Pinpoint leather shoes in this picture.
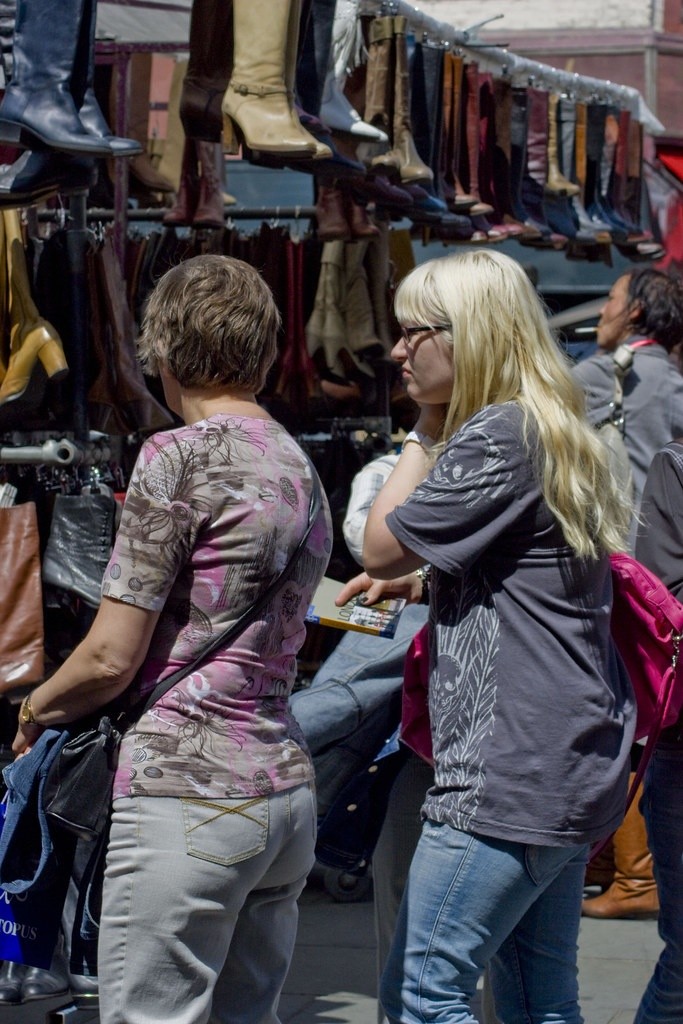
[0,961,29,1004]
[20,951,68,1002]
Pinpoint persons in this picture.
[14,253,336,1024]
[335,248,637,1024]
[570,265,683,495]
[635,438,683,1024]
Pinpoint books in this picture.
[306,576,407,639]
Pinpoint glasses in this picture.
[401,324,452,345]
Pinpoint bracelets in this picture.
[416,568,429,606]
[401,431,436,453]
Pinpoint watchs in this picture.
[22,697,37,724]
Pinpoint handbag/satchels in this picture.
[0,502,46,696]
[43,712,125,839]
[604,550,683,741]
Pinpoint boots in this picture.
[575,772,659,920]
[0,0,668,440]
[41,491,115,610]
